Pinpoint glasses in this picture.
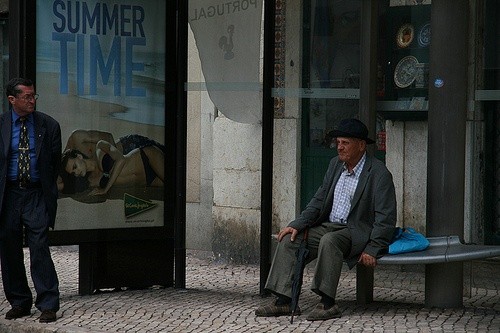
[14,93,39,100]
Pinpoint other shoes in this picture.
[305,302,342,320]
[39,311,56,322]
[255,297,301,316]
[5,306,31,319]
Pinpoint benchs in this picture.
[271,235,500,309]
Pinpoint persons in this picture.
[0,78,63,322]
[57,130,165,197]
[255,119,397,320]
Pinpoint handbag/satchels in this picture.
[385,227,430,254]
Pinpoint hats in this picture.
[334,119,376,144]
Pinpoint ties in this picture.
[17,117,32,186]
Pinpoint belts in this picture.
[7,182,40,190]
